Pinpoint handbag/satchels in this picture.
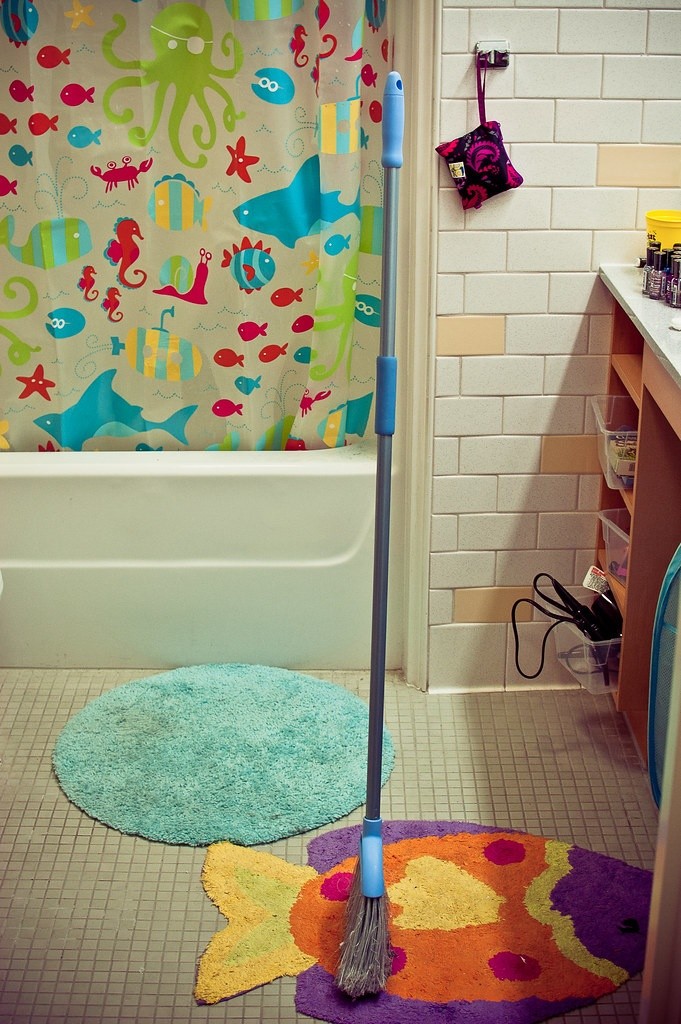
[435,51,523,210]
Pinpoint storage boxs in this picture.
[589,396,641,492]
[545,591,622,696]
[596,508,636,589]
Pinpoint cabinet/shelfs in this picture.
[594,262,681,772]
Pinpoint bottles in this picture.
[642,241,681,308]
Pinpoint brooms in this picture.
[329,69,406,998]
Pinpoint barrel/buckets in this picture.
[644,209,680,251]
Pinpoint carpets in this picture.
[50,662,655,1023]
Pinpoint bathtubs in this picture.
[1,427,407,671]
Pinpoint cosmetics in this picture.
[636,241,681,308]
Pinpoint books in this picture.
[608,435,636,476]
